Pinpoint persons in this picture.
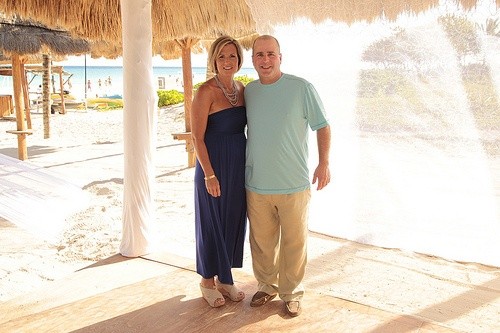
[189,33,248,308]
[38,74,186,98]
[240,33,332,317]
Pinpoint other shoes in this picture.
[250,291,272,306]
[285,301,302,317]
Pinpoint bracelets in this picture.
[203,174,216,180]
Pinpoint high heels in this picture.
[216,278,245,302]
[199,283,225,307]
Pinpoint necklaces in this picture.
[213,73,240,106]
